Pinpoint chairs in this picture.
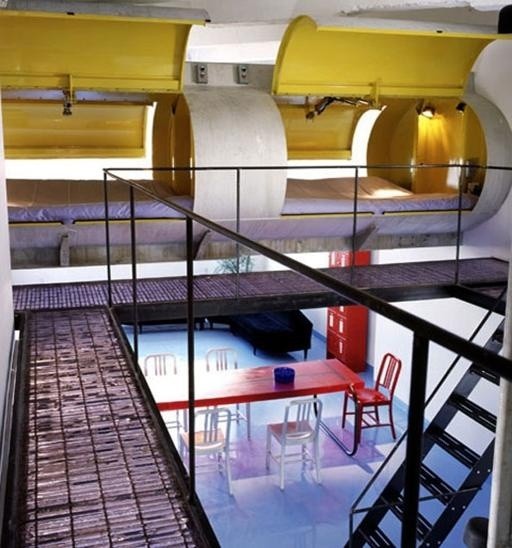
[143,347,402,496]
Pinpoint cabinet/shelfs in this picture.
[326,249,371,373]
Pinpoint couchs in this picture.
[230,310,314,362]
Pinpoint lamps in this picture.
[455,102,469,114]
[415,101,436,119]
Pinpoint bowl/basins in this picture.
[274,367,295,384]
